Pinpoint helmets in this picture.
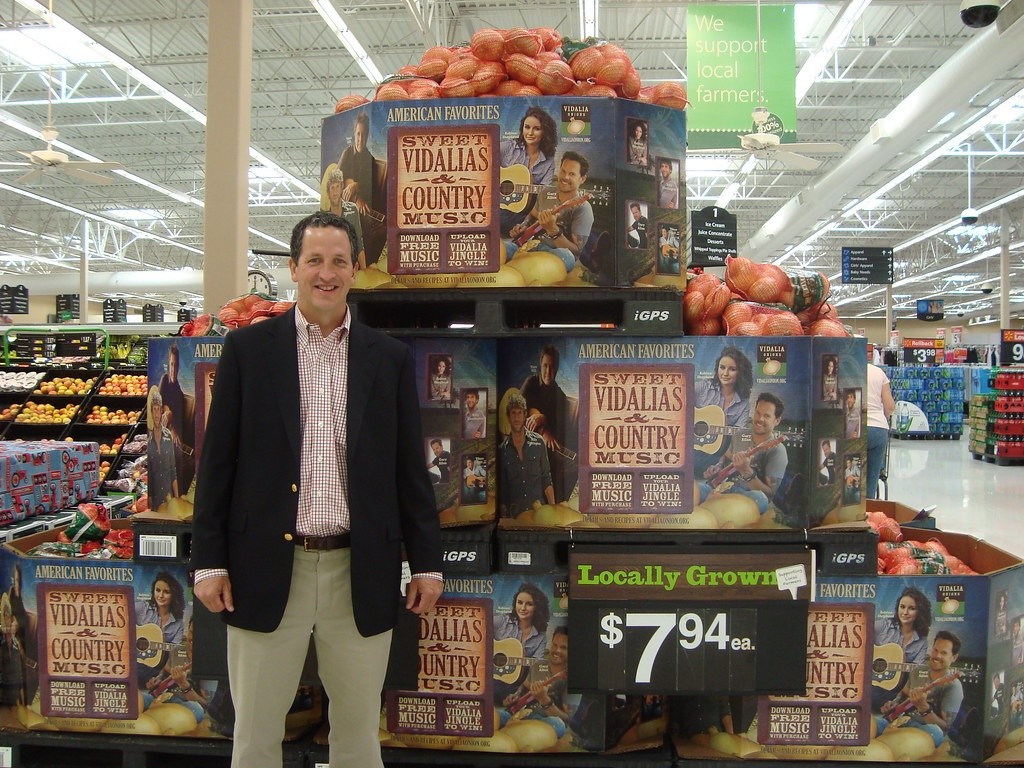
[506,393,528,417]
[326,170,344,190]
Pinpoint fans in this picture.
[11,0,122,186]
[686,0,845,169]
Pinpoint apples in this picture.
[0,373,148,486]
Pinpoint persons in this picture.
[192,211,445,768]
[865,362,895,499]
[0,106,1024,768]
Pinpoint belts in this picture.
[295,532,351,552]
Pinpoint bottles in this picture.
[896,403,901,428]
[901,403,909,429]
[882,366,965,433]
[969,366,1024,455]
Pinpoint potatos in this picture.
[180,293,296,336]
[683,256,849,339]
[861,510,982,575]
[333,29,685,108]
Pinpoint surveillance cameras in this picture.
[961,208,979,225]
[980,283,993,293]
[959,0,1001,28]
[957,311,964,317]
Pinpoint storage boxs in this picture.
[0,97,1024,768]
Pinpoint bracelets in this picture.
[548,229,562,240]
[919,705,932,716]
[541,699,554,708]
[181,684,192,693]
[742,470,757,482]
